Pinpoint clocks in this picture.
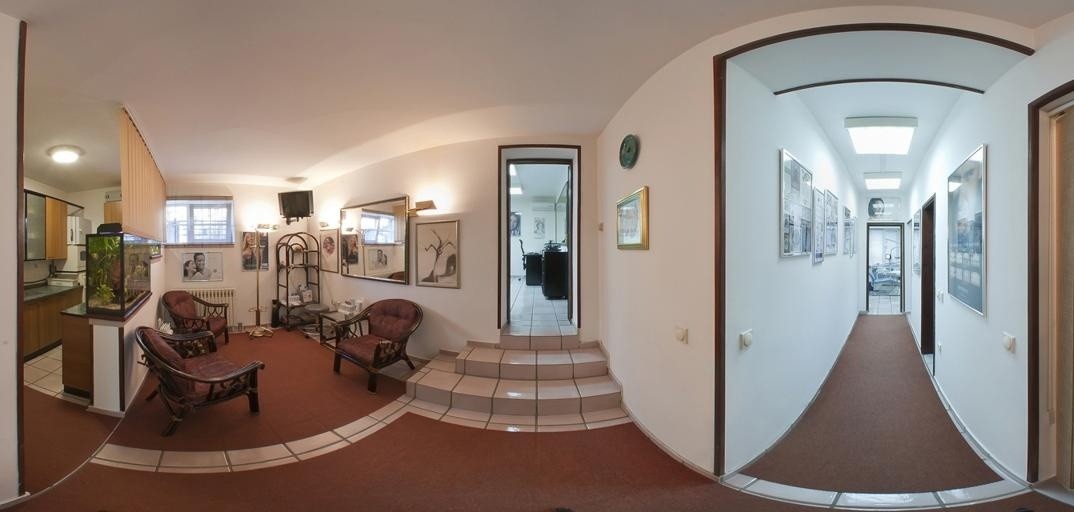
[618,135,638,169]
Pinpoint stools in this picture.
[300,303,329,339]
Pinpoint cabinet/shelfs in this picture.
[525,250,567,299]
[243,230,320,340]
[22,189,93,400]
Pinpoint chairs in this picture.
[333,298,423,394]
[134,292,265,437]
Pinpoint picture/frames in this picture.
[362,244,404,277]
[945,143,986,319]
[779,148,855,266]
[910,209,921,280]
[318,229,338,273]
[338,195,409,285]
[615,185,649,251]
[180,251,223,282]
[413,219,460,289]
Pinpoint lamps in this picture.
[509,164,522,195]
[408,200,436,218]
[843,116,918,190]
[46,145,83,164]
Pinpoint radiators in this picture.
[170,288,235,331]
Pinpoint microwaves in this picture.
[53,245,87,273]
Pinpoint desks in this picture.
[319,310,356,351]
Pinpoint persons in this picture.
[370,249,392,268]
[241,233,261,268]
[183,253,213,280]
[342,235,361,265]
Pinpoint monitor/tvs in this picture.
[278,190,314,219]
